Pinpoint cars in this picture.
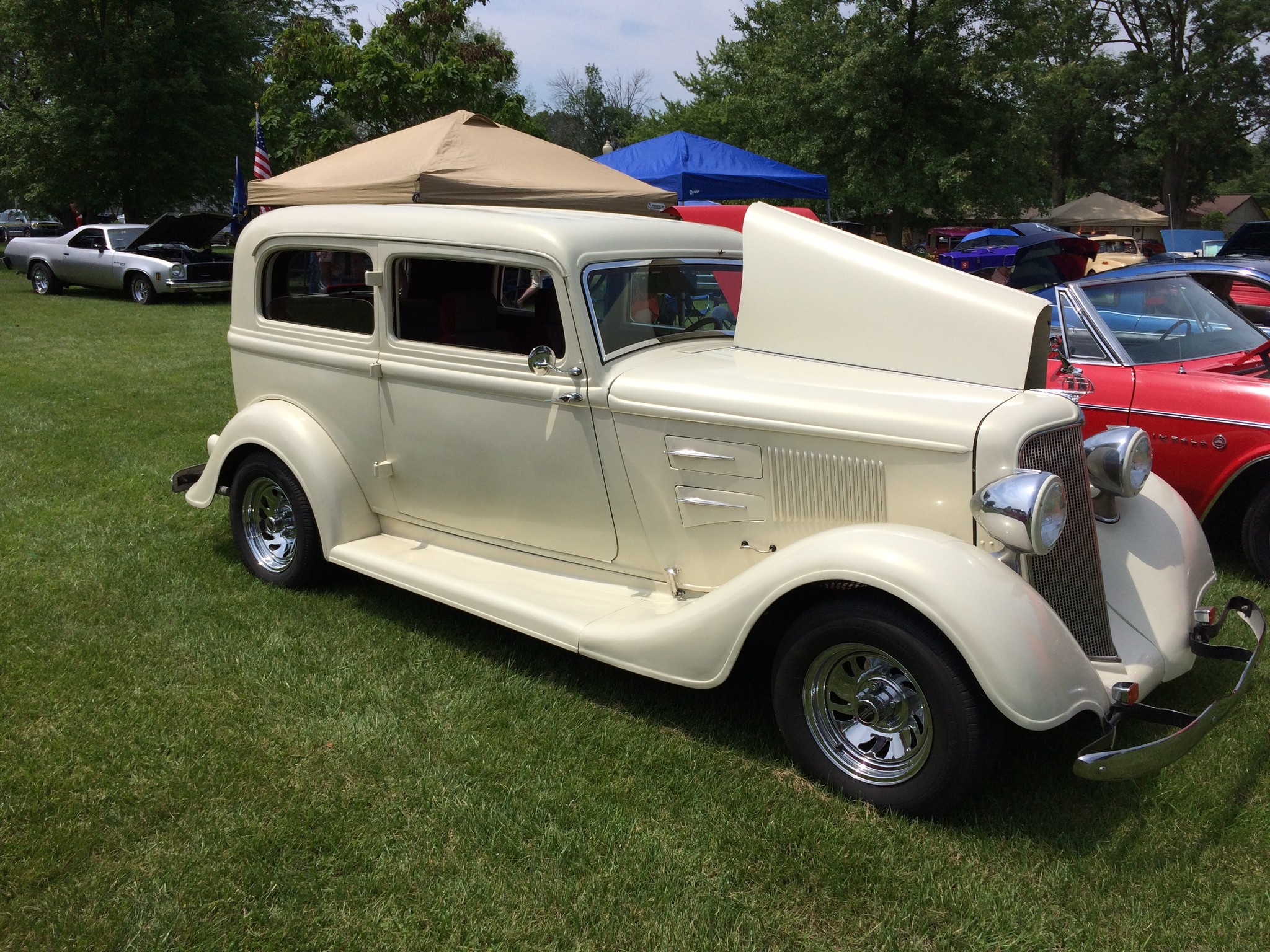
[0,204,232,304]
[167,206,1264,809]
[657,206,1270,577]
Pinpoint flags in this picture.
[229,159,252,237]
[253,112,273,214]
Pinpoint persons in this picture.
[709,293,735,328]
[516,269,557,314]
[631,267,692,325]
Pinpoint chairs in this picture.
[973,272,990,280]
[591,284,732,330]
[89,236,106,250]
[438,286,507,353]
[533,289,566,362]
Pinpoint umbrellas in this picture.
[949,227,1020,251]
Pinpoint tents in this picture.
[1029,191,1169,251]
[247,107,681,221]
[590,129,834,225]
